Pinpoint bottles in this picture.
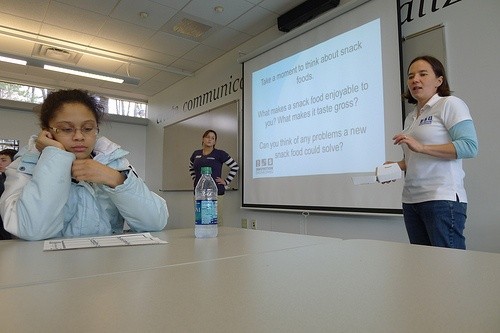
[195,167,218,239]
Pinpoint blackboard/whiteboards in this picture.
[157,98,239,192]
[402,21,448,117]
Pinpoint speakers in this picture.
[277,0,340,32]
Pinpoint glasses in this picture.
[45,124,100,137]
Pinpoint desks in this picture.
[0,227,500,333]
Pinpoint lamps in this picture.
[44,64,124,83]
[0,56,27,65]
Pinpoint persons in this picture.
[375,56,479,250]
[0,89,169,241]
[189,130,239,227]
[0,149,21,240]
